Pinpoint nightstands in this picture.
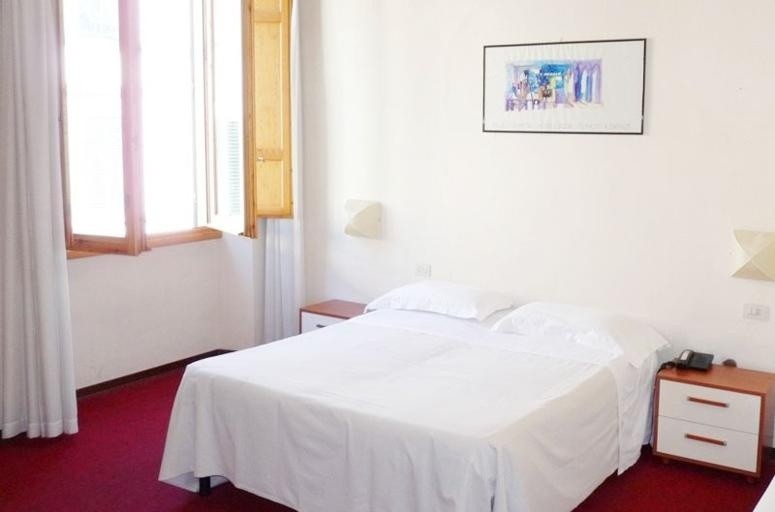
[299,299,367,332]
[653,358,774,484]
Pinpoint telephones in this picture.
[676,350,713,371]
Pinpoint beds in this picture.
[174,282,659,510]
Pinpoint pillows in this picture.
[366,279,665,367]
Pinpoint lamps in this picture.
[342,197,383,239]
[724,229,775,280]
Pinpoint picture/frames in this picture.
[482,38,646,135]
